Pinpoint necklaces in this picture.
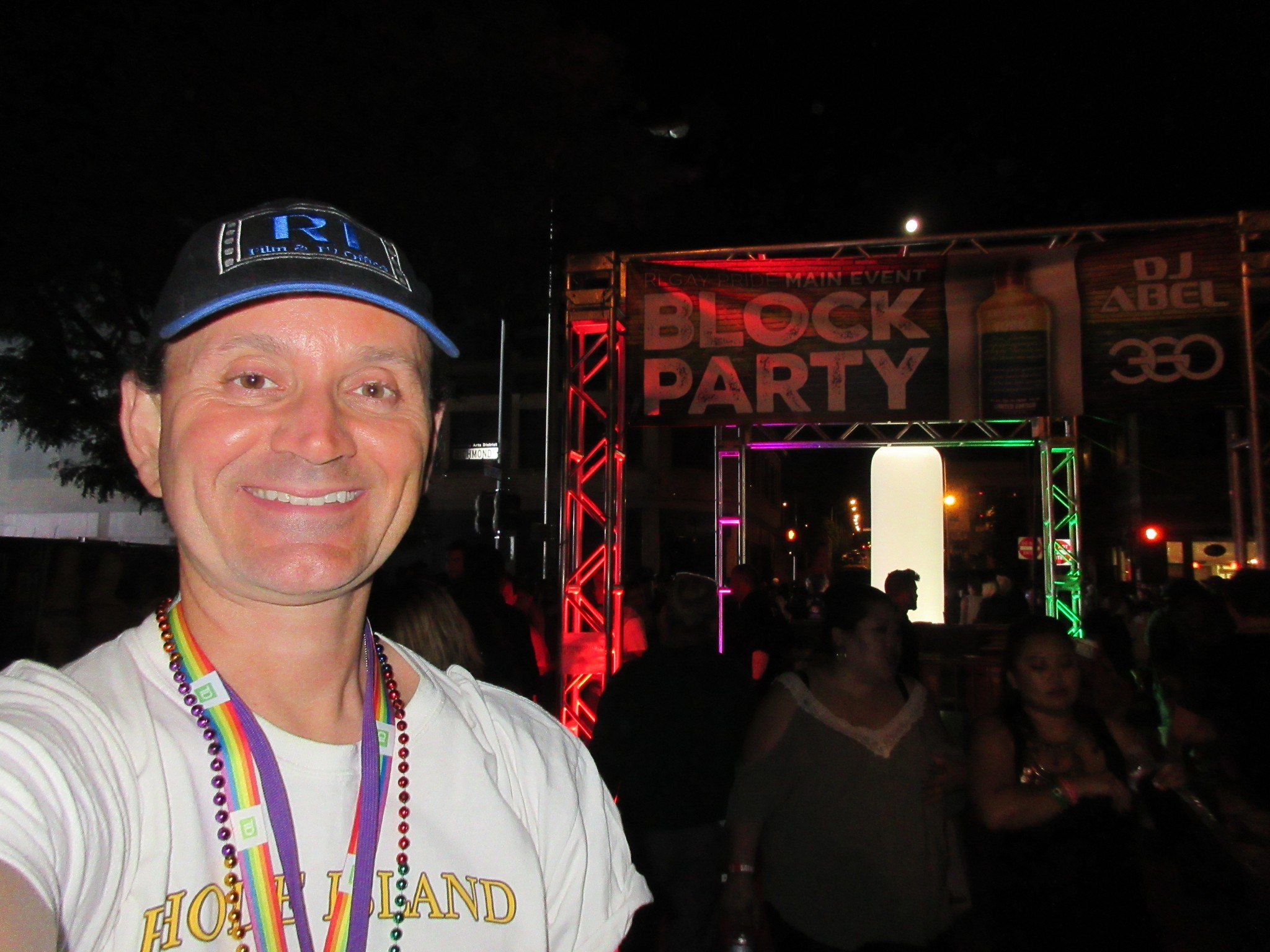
[1029,726,1084,768]
[151,590,412,952]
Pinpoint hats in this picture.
[154,197,460,360]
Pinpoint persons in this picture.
[0,195,659,952]
[1169,561,1270,952]
[716,584,980,952]
[351,532,1238,754]
[963,614,1197,952]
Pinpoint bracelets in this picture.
[725,861,758,877]
[1038,770,1079,810]
